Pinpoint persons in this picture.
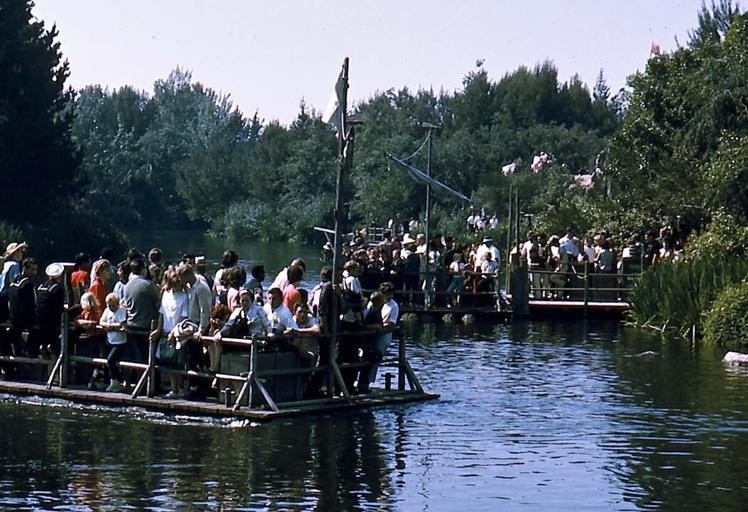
[500,149,613,199]
[1,238,402,406]
[340,206,700,310]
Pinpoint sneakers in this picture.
[87,378,207,402]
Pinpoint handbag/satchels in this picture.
[220,317,250,339]
[155,338,179,368]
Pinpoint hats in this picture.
[45,262,65,279]
[481,235,493,246]
[401,237,416,245]
[3,242,30,261]
